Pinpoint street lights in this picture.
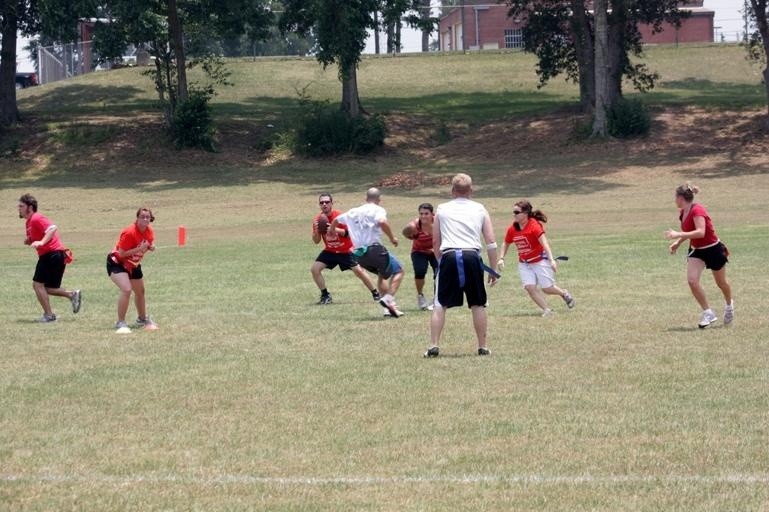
[714,26,722,42]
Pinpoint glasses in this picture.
[513,211,523,215]
[320,201,330,204]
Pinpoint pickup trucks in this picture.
[15,72,38,90]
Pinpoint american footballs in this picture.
[403,224,417,235]
[318,213,329,233]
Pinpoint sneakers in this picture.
[116,320,130,329]
[373,292,434,318]
[478,348,491,355]
[71,289,81,313]
[135,317,159,327]
[698,313,718,328]
[317,295,332,304]
[723,300,734,324]
[561,289,575,308]
[424,347,439,358]
[35,313,57,323]
[542,308,555,317]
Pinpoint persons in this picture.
[328,187,406,318]
[402,202,441,312]
[310,192,382,306]
[664,182,736,329]
[17,193,82,322]
[421,172,500,359]
[105,207,160,334]
[495,200,576,318]
